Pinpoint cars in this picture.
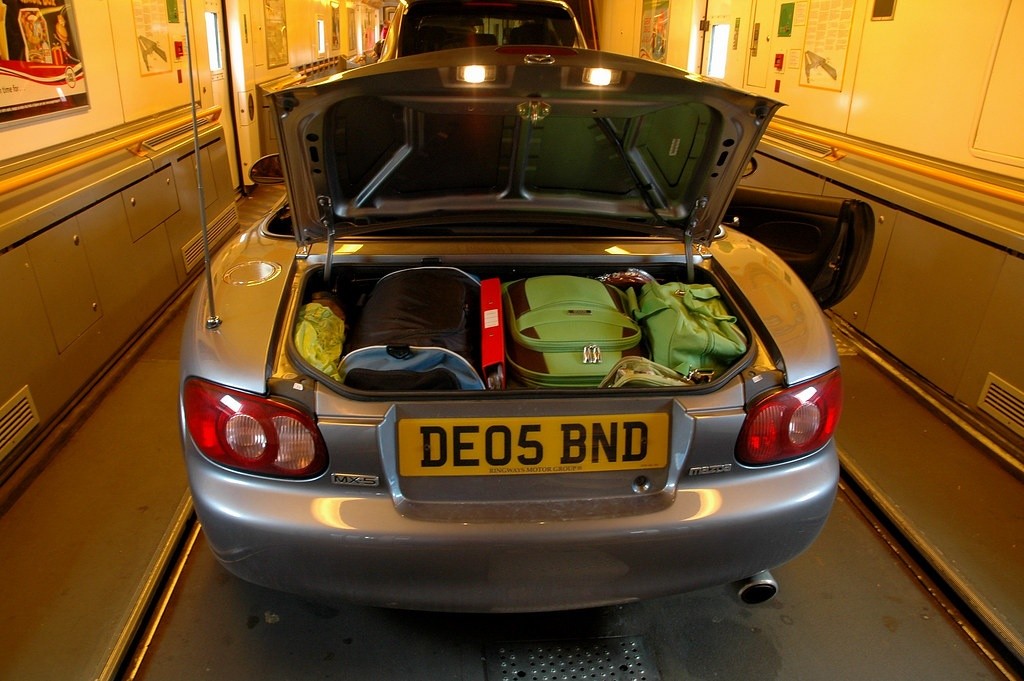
[178,44,875,647]
[374,1,588,89]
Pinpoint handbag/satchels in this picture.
[337,264,486,391]
[595,356,697,388]
[623,277,749,384]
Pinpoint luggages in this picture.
[501,275,650,389]
